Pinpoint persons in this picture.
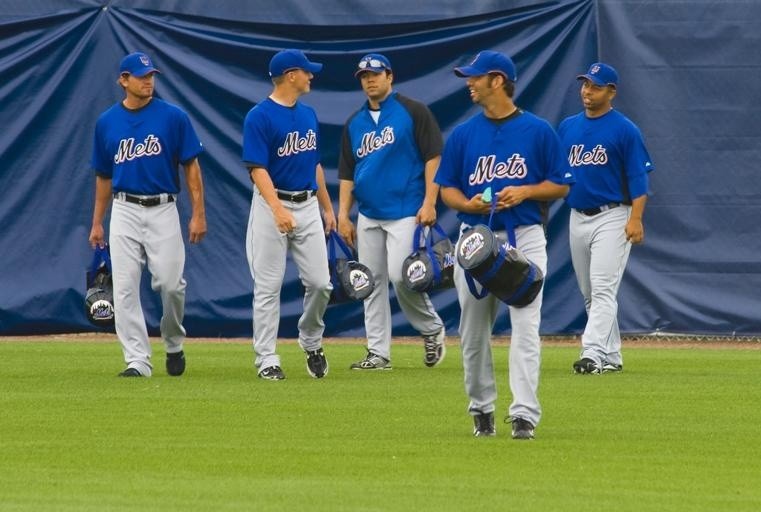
[433,50,576,441]
[243,49,336,381]
[338,53,445,370]
[556,63,655,373]
[89,54,208,377]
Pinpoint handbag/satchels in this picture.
[84,245,114,326]
[457,195,544,308]
[303,231,375,305]
[401,222,456,292]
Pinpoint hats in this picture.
[454,51,517,82]
[268,49,323,76]
[354,54,392,78]
[119,52,161,78]
[578,62,620,89]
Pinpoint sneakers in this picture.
[298,339,329,379]
[574,358,601,374]
[260,366,285,380]
[470,408,496,438]
[424,327,445,366]
[350,352,393,371]
[118,368,141,377]
[504,415,534,439]
[166,350,186,376]
[603,362,622,372]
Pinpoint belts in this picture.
[574,201,621,216]
[275,190,316,203]
[114,193,174,207]
[488,225,519,231]
